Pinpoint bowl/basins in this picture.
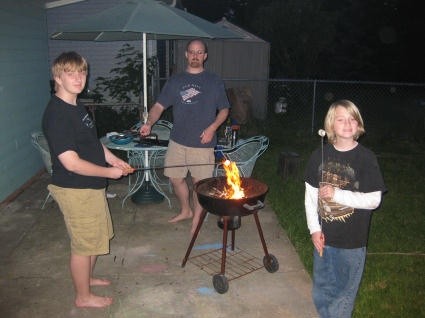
[110,134,133,144]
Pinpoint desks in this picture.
[98,136,172,209]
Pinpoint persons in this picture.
[302,99,387,318]
[42,52,134,308]
[140,39,231,236]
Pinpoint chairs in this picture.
[214,135,270,179]
[126,120,173,194]
[31,133,54,208]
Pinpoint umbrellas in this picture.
[49,0,245,182]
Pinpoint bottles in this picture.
[224,119,238,147]
